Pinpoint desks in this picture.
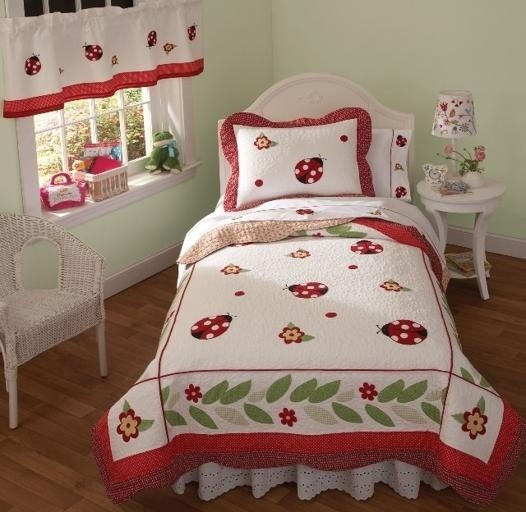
[416,175,505,301]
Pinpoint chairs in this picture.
[0,213,108,427]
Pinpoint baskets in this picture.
[71,164,128,203]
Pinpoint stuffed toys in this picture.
[143,129,184,176]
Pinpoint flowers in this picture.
[436,144,487,175]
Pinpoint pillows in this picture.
[220,106,414,212]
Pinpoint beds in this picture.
[92,70,525,508]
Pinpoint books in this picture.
[445,250,493,277]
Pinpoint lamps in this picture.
[432,91,477,182]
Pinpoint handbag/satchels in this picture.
[39,172,88,211]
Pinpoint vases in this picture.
[465,172,485,187]
